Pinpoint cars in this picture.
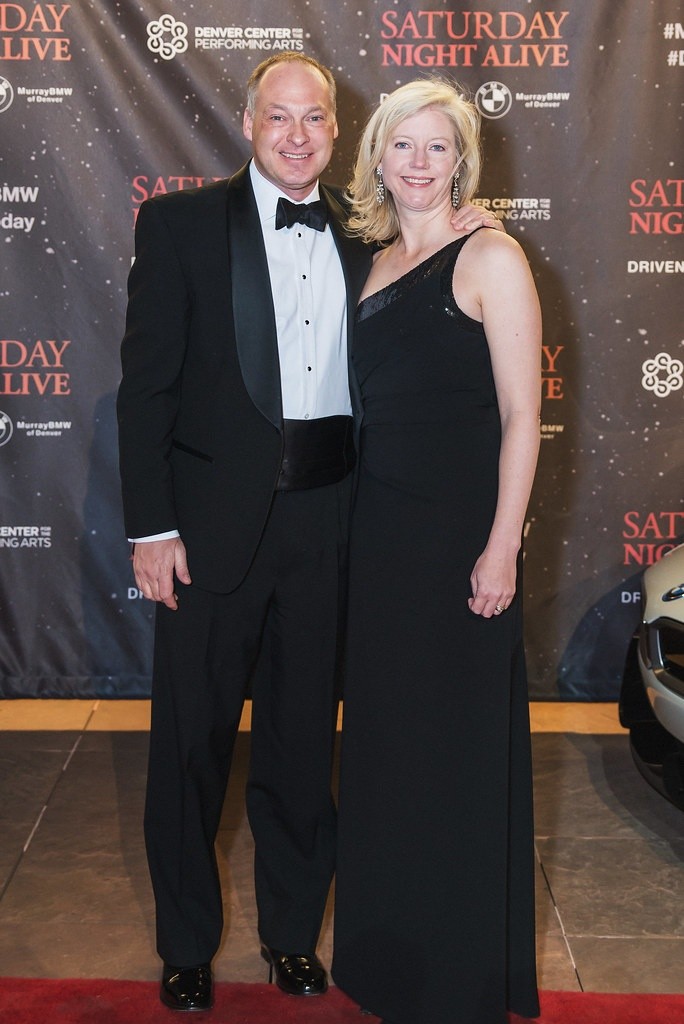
[637,542,684,744]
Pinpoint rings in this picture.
[495,605,504,612]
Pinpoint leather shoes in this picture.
[160,961,215,1012]
[261,943,329,996]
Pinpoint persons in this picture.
[116,54,507,1015]
[331,69,543,1024]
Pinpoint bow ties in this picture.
[275,196,327,232]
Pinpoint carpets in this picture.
[0,980,683,1023]
